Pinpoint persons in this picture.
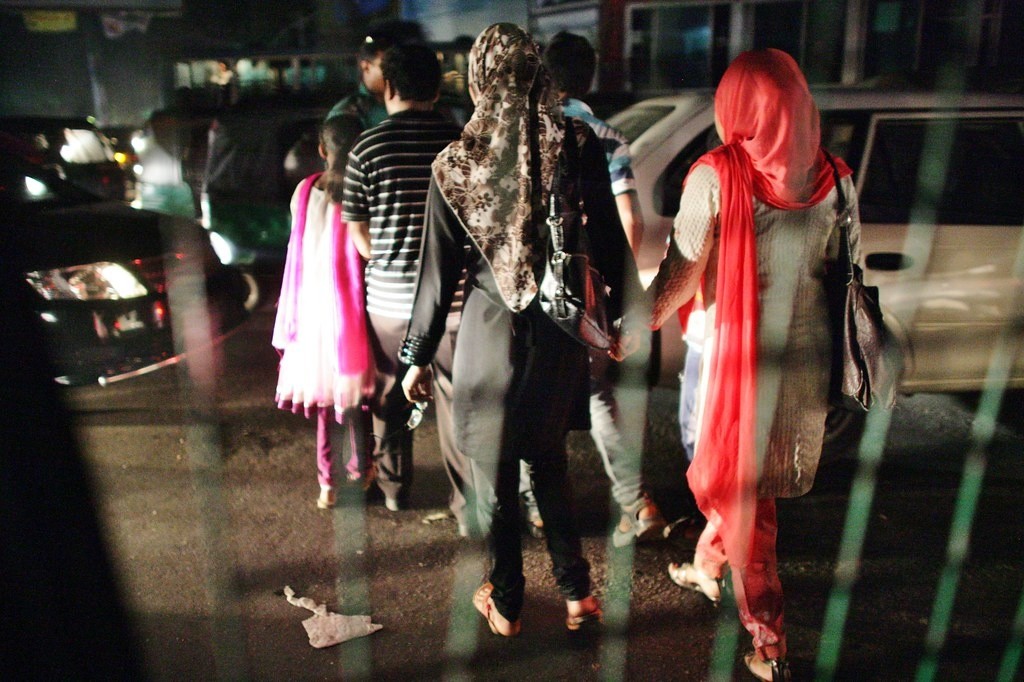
[435,70,474,126]
[527,31,664,551]
[610,46,862,682]
[399,24,644,643]
[321,39,393,128]
[342,46,476,541]
[273,112,374,511]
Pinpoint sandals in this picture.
[614,496,664,548]
[522,499,546,538]
[743,652,792,682]
[669,562,727,602]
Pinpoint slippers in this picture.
[564,606,603,630]
[474,582,520,637]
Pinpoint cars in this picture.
[600,75,1024,473]
[0,127,260,395]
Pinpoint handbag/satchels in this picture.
[822,149,898,413]
[540,116,615,350]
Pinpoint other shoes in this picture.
[348,473,373,492]
[317,487,336,510]
[376,478,407,511]
